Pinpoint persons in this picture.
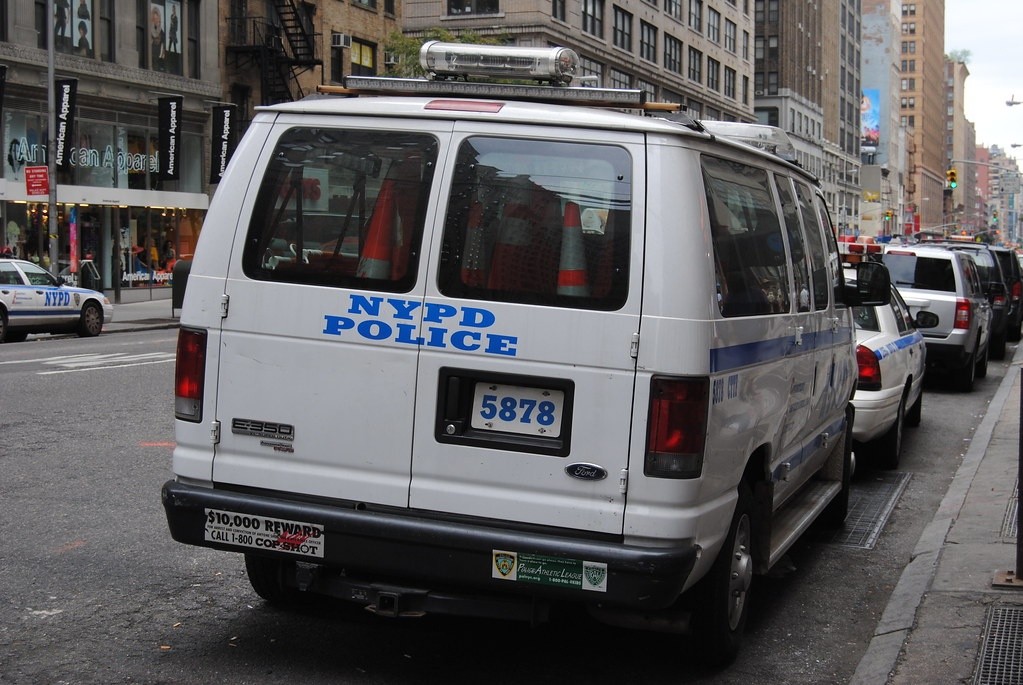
[164,240,176,271]
[151,239,161,270]
[53,0,69,44]
[168,5,177,54]
[4,138,26,182]
[131,245,146,274]
[78,21,89,49]
[151,7,164,58]
[77,0,89,19]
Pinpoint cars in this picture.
[837,261,939,472]
[0,258,114,343]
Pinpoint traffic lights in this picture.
[946,168,958,188]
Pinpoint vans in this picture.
[158,39,894,674]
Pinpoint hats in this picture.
[762,279,770,283]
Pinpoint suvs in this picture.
[882,236,1023,392]
